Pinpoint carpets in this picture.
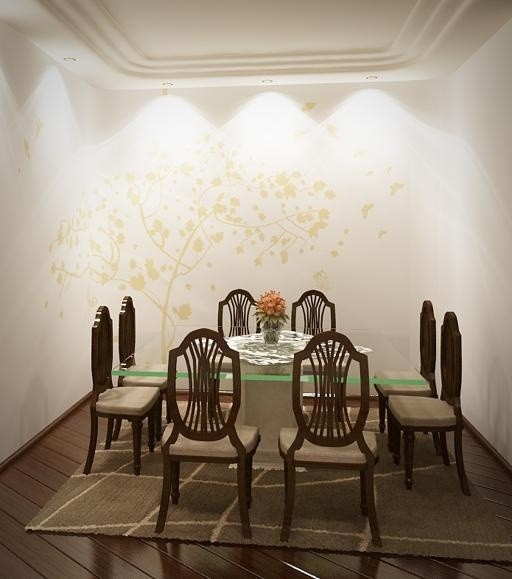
[25,399,512,563]
